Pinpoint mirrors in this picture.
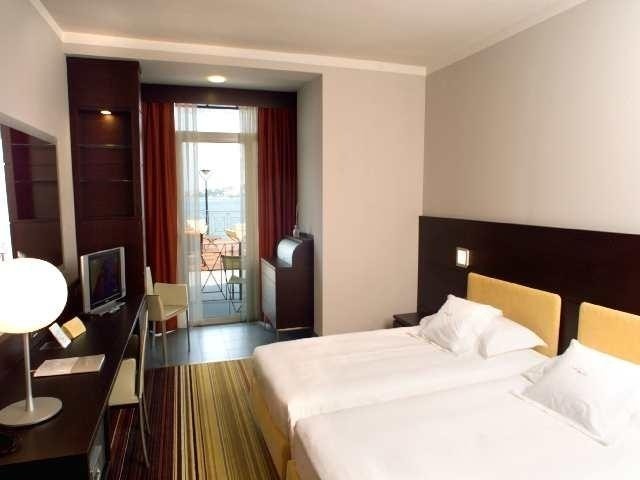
[2,112,64,277]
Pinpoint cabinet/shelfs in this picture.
[0,285,149,480]
[261,235,314,343]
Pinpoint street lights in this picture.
[200,170,212,235]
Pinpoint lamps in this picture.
[0,257,68,428]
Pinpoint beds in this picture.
[248,273,640,480]
[248,273,565,480]
[291,300,640,480]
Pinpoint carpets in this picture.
[101,357,282,480]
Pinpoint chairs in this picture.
[221,254,246,314]
[109,307,151,469]
[143,267,190,368]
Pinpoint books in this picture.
[33,354,106,379]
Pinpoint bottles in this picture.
[292,223,301,238]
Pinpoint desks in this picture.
[199,236,242,300]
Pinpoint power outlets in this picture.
[455,248,469,268]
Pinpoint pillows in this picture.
[417,294,503,349]
[529,338,639,447]
[478,317,546,357]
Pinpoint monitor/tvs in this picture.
[80,247,126,316]
